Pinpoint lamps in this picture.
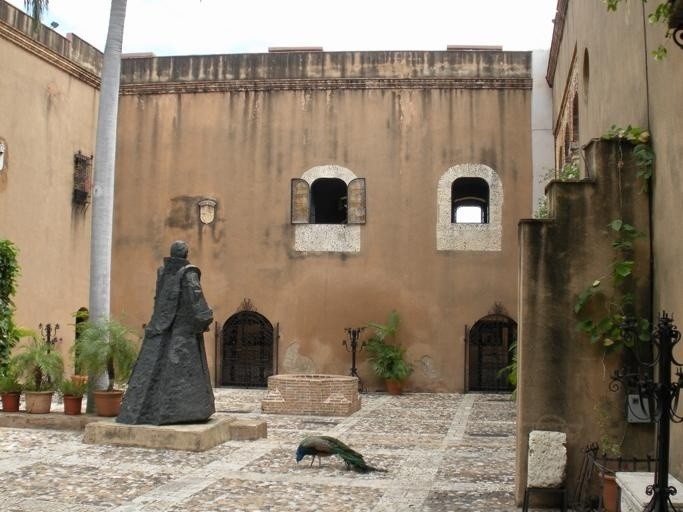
[50,21,58,30]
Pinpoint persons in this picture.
[116,240,215,425]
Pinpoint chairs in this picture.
[523,417,568,512]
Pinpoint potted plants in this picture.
[9,328,64,414]
[576,399,652,512]
[69,310,142,416]
[60,379,88,414]
[366,312,414,394]
[0,369,23,411]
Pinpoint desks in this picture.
[614,471,683,512]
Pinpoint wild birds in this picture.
[295,436,389,475]
